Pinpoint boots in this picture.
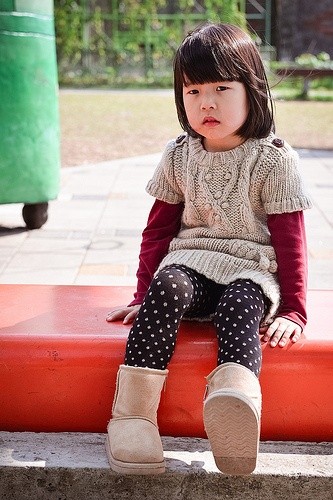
[106,365,169,475]
[203,362,261,475]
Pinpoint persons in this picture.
[105,22,311,475]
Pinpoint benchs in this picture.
[0,284,333,442]
[270,67,333,97]
